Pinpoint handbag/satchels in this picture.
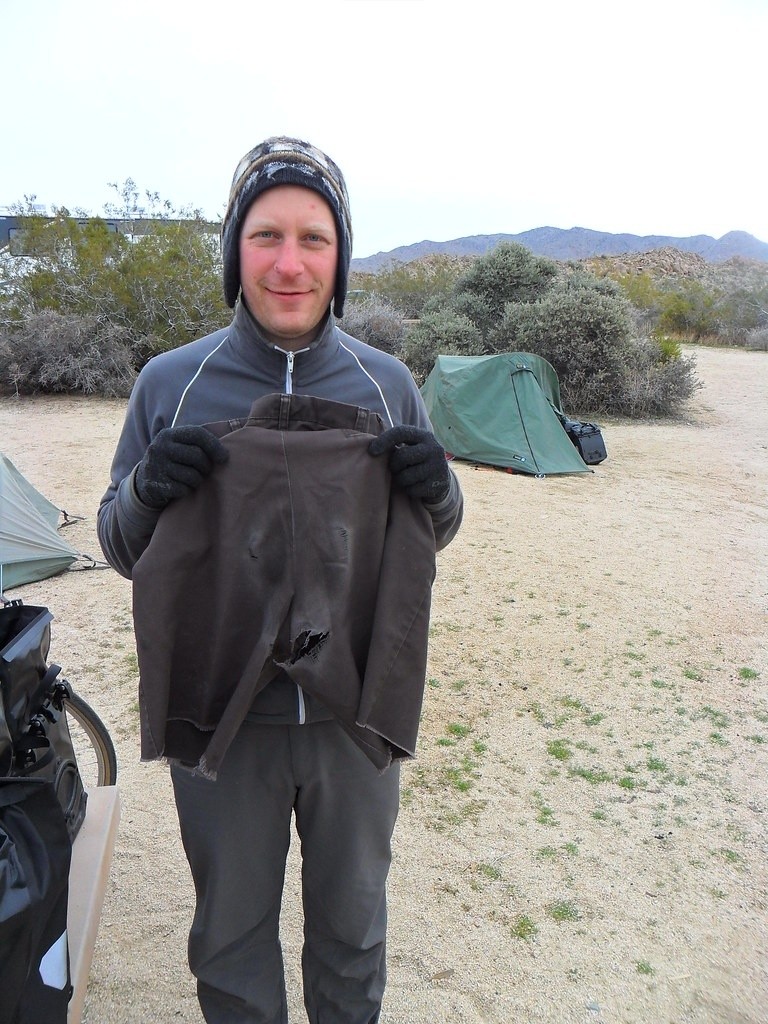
[0,600,88,844]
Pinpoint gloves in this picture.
[368,423,450,504]
[135,425,230,509]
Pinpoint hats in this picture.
[221,138,352,319]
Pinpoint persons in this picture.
[96,136,464,1024]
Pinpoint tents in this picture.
[419,351,594,476]
[0,453,79,591]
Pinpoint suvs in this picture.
[0,597,121,1024]
